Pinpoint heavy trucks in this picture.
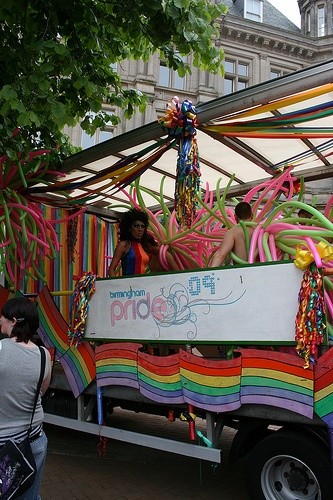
[0,188,333,500]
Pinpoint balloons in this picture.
[107,166,333,347]
[0,127,92,296]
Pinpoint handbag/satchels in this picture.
[0,438,37,500]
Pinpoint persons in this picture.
[203,200,265,269]
[108,206,152,276]
[0,296,52,499]
